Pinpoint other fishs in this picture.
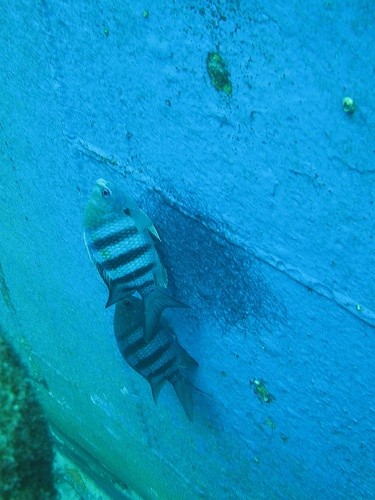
[113,296,214,424]
[81,179,195,345]
[206,51,234,96]
